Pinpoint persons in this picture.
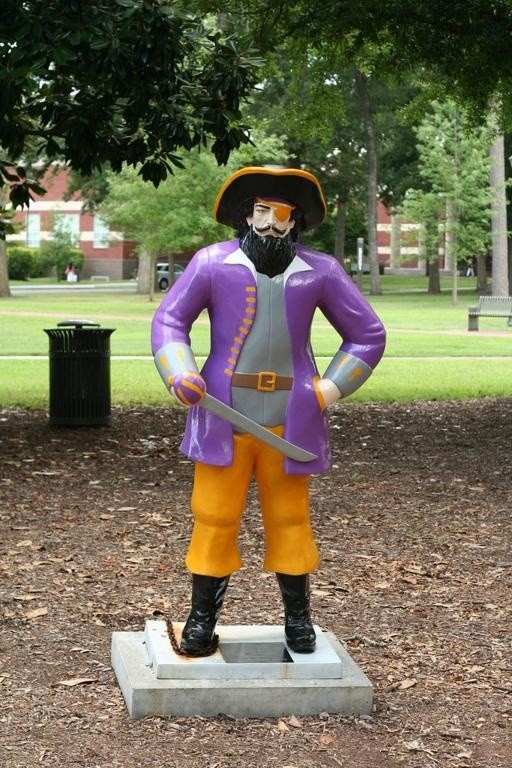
[466,259,476,278]
[148,161,389,657]
[64,261,75,284]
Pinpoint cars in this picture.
[156,262,185,289]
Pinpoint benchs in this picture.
[468,296,512,331]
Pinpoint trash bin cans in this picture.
[42,319,116,428]
